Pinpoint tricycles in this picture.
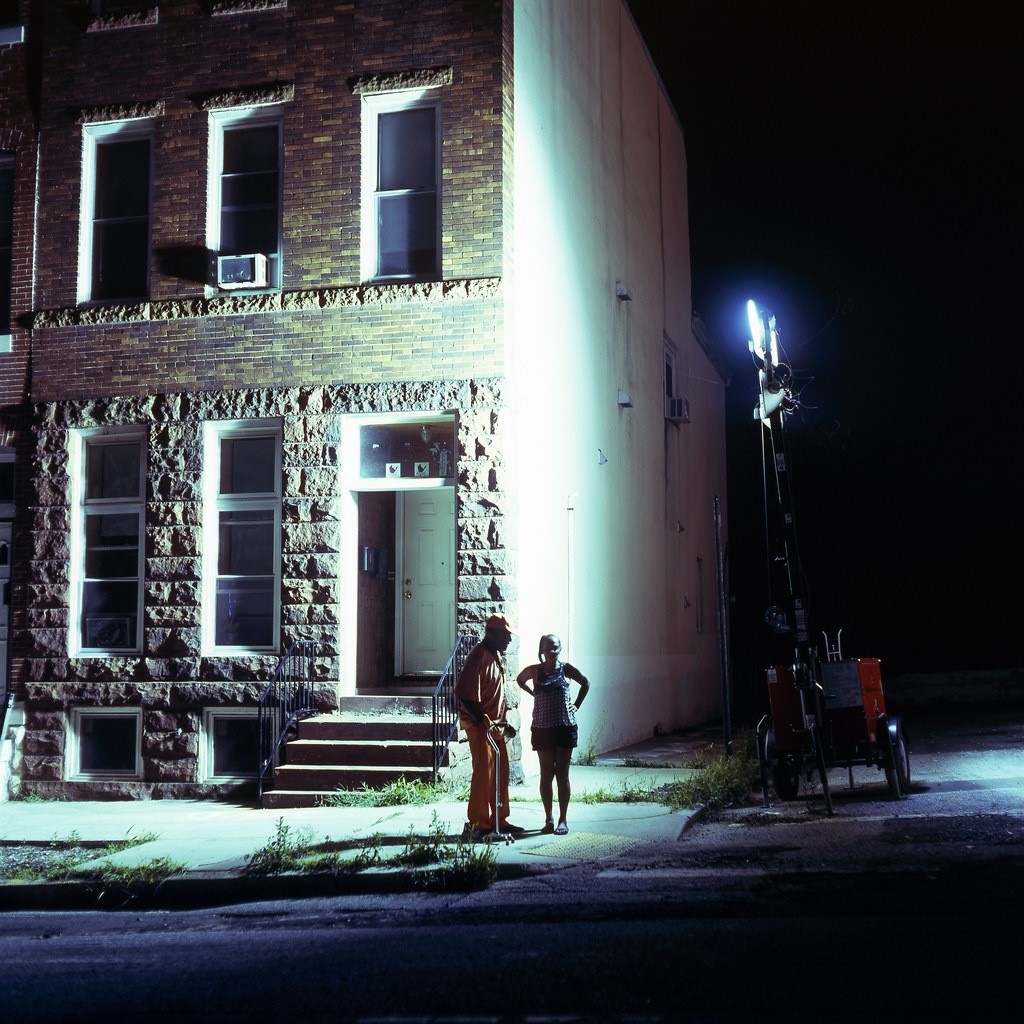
[755,626,915,801]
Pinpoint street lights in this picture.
[746,298,831,797]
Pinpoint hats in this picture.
[486,613,515,634]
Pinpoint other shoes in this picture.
[492,823,525,835]
[462,823,501,841]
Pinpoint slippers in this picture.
[542,822,553,833]
[555,823,569,835]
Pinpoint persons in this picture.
[516,634,591,833]
[454,613,524,838]
